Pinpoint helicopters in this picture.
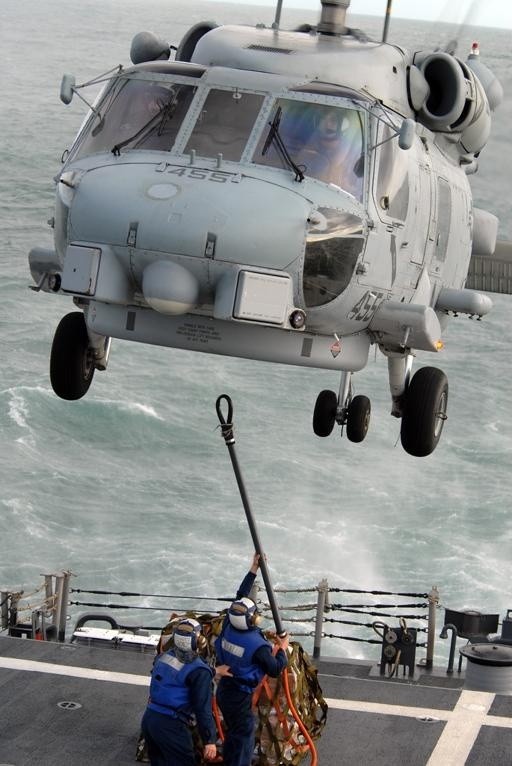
[27,0,512,458]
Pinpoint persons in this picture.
[297,110,353,177]
[215,544,289,766]
[141,617,218,765]
[110,85,173,143]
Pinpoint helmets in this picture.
[228,597,260,631]
[173,619,203,652]
